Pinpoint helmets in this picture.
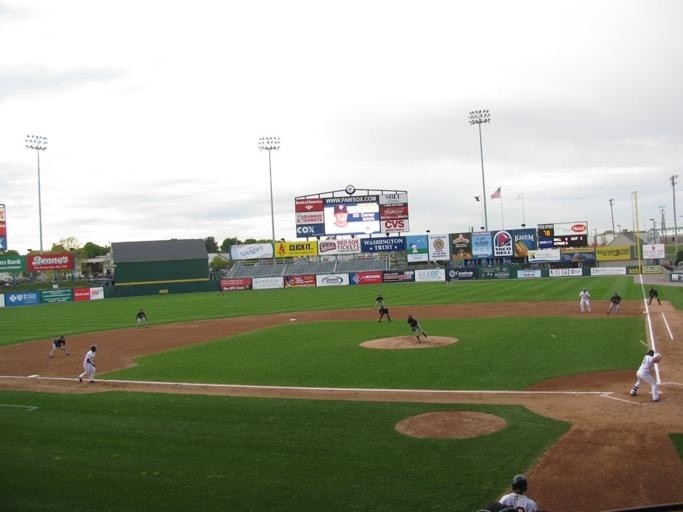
[511,474,528,492]
[647,350,654,356]
[91,345,97,351]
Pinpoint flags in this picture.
[491,186,501,199]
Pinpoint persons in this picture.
[78,344,98,384]
[378,305,392,322]
[578,288,591,313]
[49,335,68,358]
[331,204,349,229]
[136,308,149,329]
[629,348,662,402]
[374,294,384,308]
[648,287,661,306]
[407,315,428,343]
[607,293,621,315]
[498,474,538,512]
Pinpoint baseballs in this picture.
[289,318,296,322]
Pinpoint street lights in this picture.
[467,107,491,232]
[22,132,47,253]
[257,135,281,243]
[608,198,616,237]
[615,225,621,233]
[669,173,678,243]
[593,229,598,247]
[649,217,656,243]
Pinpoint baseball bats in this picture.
[640,340,652,351]
